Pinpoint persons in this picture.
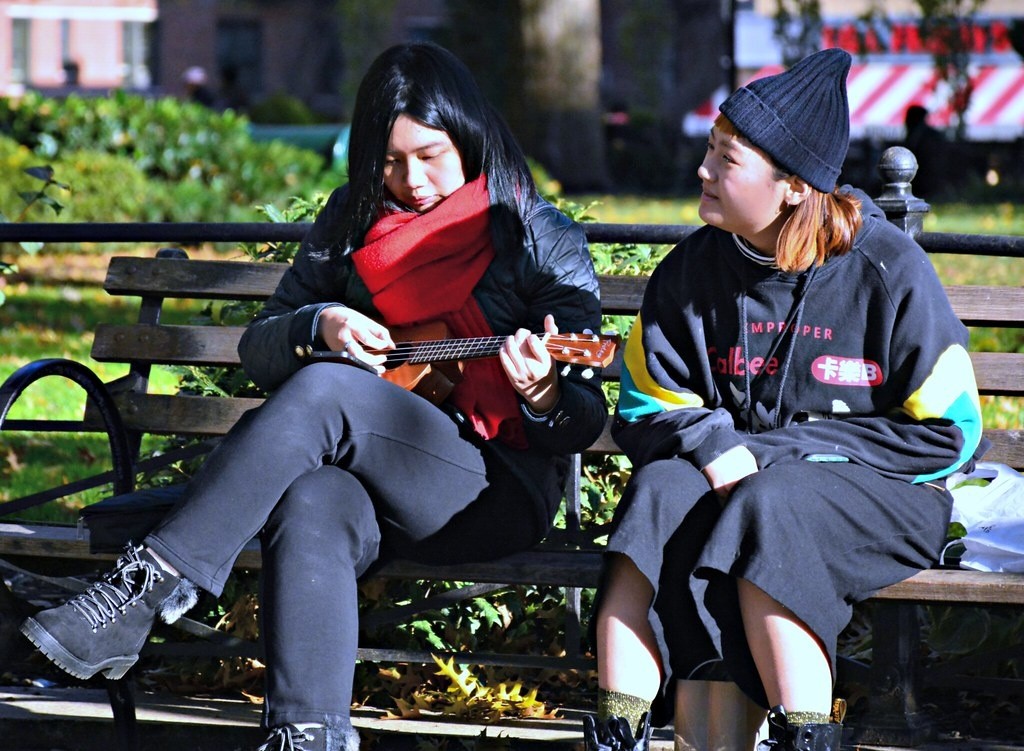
[62,60,78,85]
[20,41,607,751]
[875,105,951,159]
[584,48,982,751]
[184,68,214,106]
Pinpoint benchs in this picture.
[1,251,1024,751]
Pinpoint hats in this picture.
[719,48,852,192]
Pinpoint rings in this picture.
[344,340,355,352]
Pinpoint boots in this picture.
[19,540,199,681]
[257,715,361,751]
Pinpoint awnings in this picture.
[684,61,1024,143]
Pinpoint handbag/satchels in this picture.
[940,462,1024,573]
[77,483,188,554]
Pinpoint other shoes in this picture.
[582,711,651,751]
[757,698,847,751]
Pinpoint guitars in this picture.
[365,320,618,391]
